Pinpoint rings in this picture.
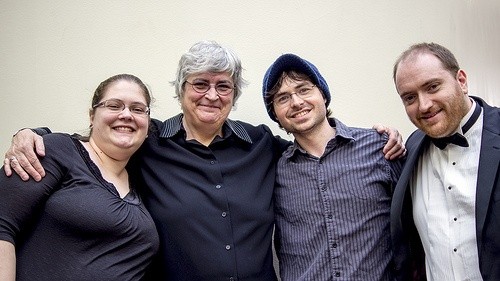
[10,156,15,162]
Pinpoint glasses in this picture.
[185,80,236,96]
[271,85,316,106]
[93,97,150,119]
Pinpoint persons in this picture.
[4,40,406,281]
[0,74,160,281]
[392,42,500,281]
[262,54,405,281]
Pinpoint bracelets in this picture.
[13,127,37,137]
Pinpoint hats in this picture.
[262,53,331,123]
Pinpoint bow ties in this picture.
[431,133,468,150]
